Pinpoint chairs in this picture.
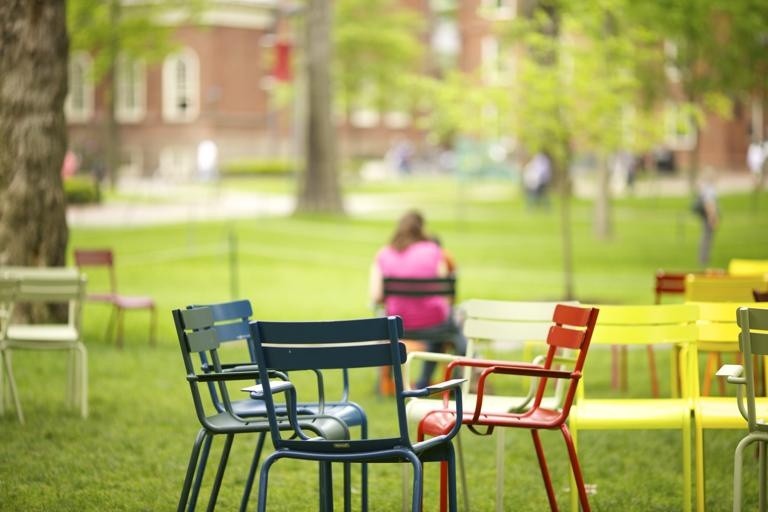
[403,299,577,512]
[688,302,768,512]
[381,276,466,403]
[571,302,695,512]
[69,246,159,349]
[251,320,466,512]
[187,300,369,512]
[729,258,768,302]
[649,272,732,402]
[172,307,353,512]
[416,305,600,512]
[731,309,768,512]
[0,264,88,423]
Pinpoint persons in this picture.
[693,162,721,268]
[369,209,466,401]
[745,135,768,196]
